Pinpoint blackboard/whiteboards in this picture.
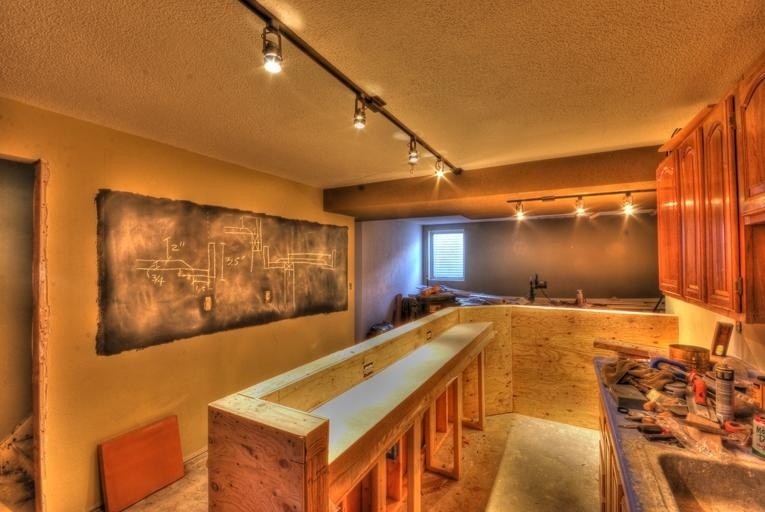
[96,189,348,355]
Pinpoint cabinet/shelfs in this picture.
[598,393,631,511]
[654,49,765,326]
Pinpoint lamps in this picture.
[261,20,463,179]
[514,191,635,219]
